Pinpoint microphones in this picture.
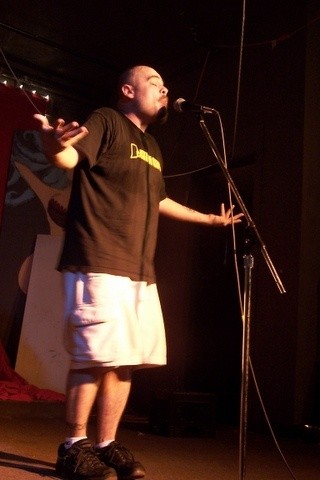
[173,97,214,114]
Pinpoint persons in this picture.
[33,64,244,479]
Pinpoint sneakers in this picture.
[96,442,146,477]
[55,439,116,480]
